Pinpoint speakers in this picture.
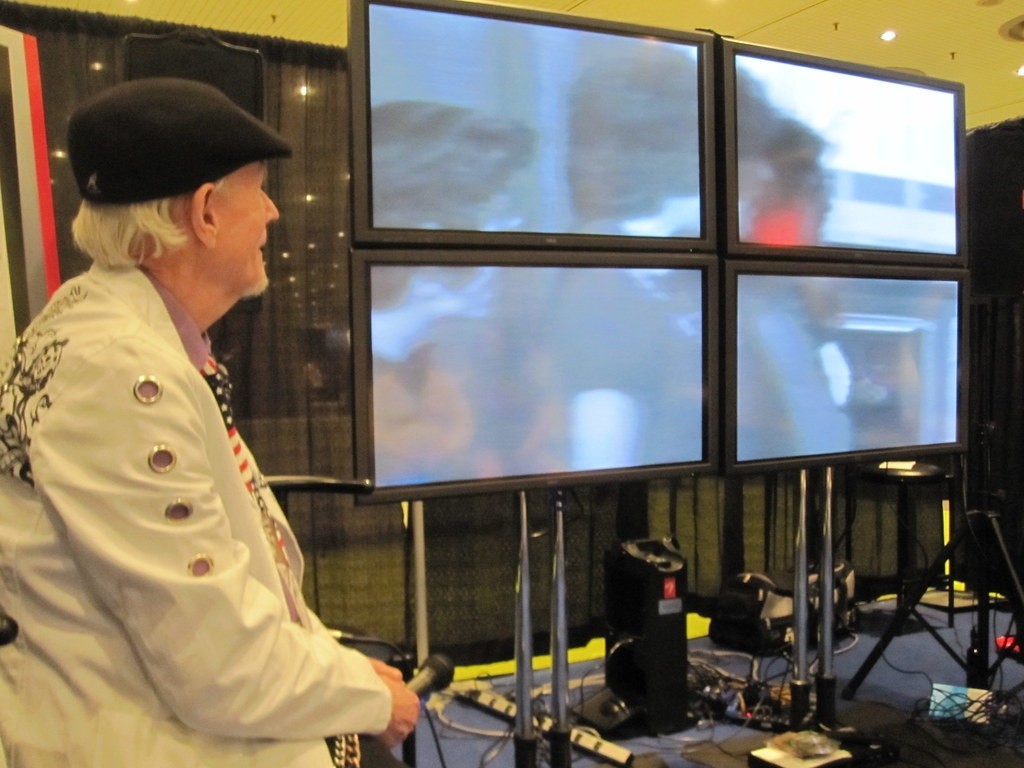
[602,533,692,731]
[119,27,266,314]
[965,122,1023,300]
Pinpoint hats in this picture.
[67,77,295,204]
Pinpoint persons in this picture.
[0,78,422,768]
[370,48,860,495]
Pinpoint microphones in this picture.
[405,651,459,699]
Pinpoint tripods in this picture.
[834,293,1024,691]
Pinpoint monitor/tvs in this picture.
[721,33,966,269]
[350,0,719,255]
[351,251,723,508]
[720,257,972,473]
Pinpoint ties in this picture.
[198,359,305,630]
